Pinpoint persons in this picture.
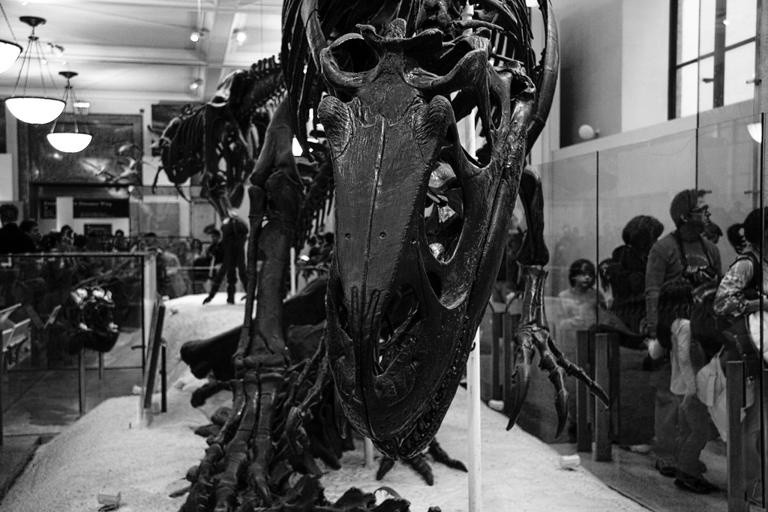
[554,187,767,505]
[0,201,221,353]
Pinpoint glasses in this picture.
[688,205,709,215]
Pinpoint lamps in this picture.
[98,491,121,512]
[561,453,581,471]
[234,29,246,43]
[190,27,209,41]
[489,399,505,410]
[579,125,599,140]
[189,79,203,90]
[0,17,93,153]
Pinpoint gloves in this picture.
[647,339,667,360]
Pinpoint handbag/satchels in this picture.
[720,316,762,376]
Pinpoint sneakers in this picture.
[620,443,726,495]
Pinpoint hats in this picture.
[671,189,712,226]
